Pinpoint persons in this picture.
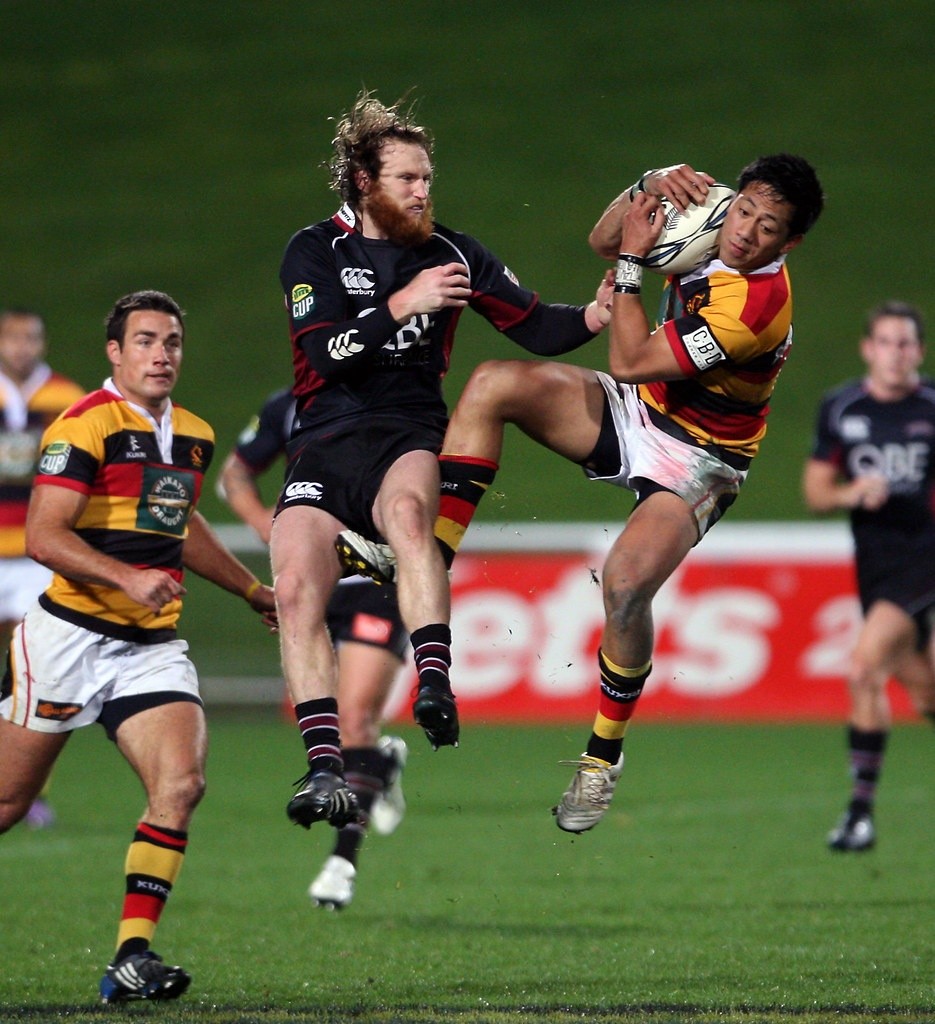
[266,98,616,830]
[801,300,935,852]
[219,386,412,908]
[0,315,92,833]
[0,291,280,1001]
[332,154,828,833]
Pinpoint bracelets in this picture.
[613,253,647,295]
[629,169,660,203]
[245,579,261,599]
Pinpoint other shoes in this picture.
[27,801,53,828]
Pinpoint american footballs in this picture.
[642,182,738,276]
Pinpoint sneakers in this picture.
[288,769,359,830]
[556,745,624,833]
[832,812,875,851]
[335,530,453,602]
[367,735,407,836]
[100,949,192,1004]
[413,688,461,752]
[309,855,357,910]
